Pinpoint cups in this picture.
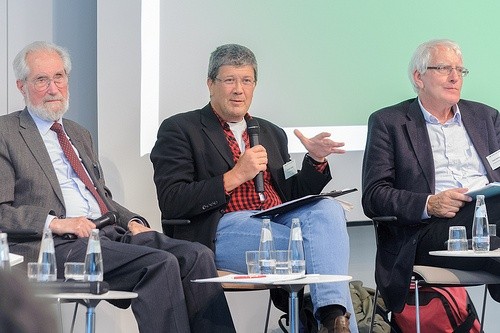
[63,262,85,282]
[246,250,271,276]
[484,225,496,236]
[270,250,292,275]
[447,226,468,252]
[28,262,51,283]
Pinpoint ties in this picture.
[50,121,109,216]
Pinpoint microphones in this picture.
[65,211,118,239]
[441,235,500,250]
[34,280,110,295]
[247,118,265,192]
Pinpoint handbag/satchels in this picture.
[269,281,391,333]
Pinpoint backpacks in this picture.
[391,272,482,333]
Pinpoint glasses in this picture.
[22,75,70,91]
[426,64,469,77]
[213,77,256,87]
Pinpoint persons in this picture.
[361,38,500,314]
[0,40,237,333]
[150,44,358,333]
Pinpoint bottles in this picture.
[0,232,11,275]
[472,194,490,252]
[83,228,103,282]
[37,229,58,282]
[288,217,305,277]
[258,216,275,274]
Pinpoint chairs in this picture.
[161,220,272,333]
[367,215,500,333]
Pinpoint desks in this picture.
[429,250,500,257]
[272,274,353,333]
[37,289,138,333]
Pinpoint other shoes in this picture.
[320,311,351,333]
